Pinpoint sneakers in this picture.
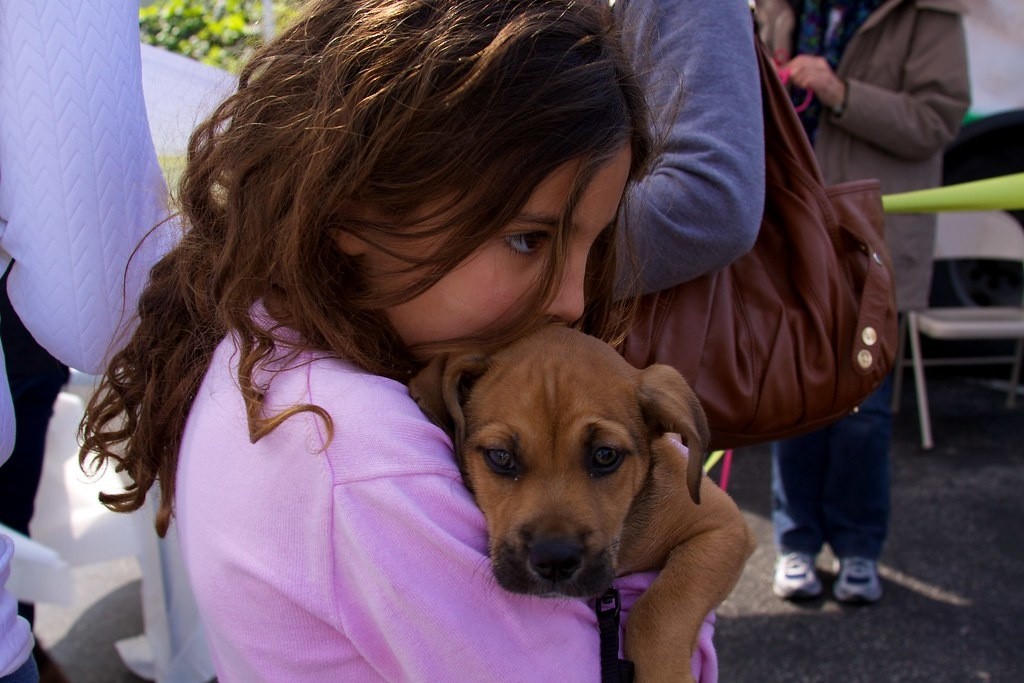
[833,553,882,604]
[772,542,822,598]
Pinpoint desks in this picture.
[884,173,1024,210]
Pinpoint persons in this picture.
[750,0,972,601]
[0,262,70,683]
[603,0,765,301]
[79,0,717,683]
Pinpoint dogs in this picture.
[405,327,758,683]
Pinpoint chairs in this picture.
[880,210,1024,451]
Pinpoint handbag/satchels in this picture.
[592,16,900,447]
